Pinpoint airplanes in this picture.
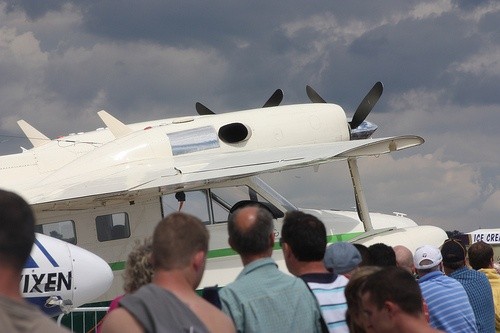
[0,75,453,330]
[1,214,120,325]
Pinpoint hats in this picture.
[413,246,443,269]
[440,238,467,263]
[323,241,363,275]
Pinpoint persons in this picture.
[413,244,478,333]
[0,189,75,333]
[344,265,383,333]
[441,238,495,333]
[280,210,351,333]
[202,202,322,333]
[393,245,416,275]
[323,242,363,283]
[352,243,378,264]
[99,238,157,333]
[468,241,500,333]
[361,265,444,333]
[101,211,236,332]
[368,242,396,266]
